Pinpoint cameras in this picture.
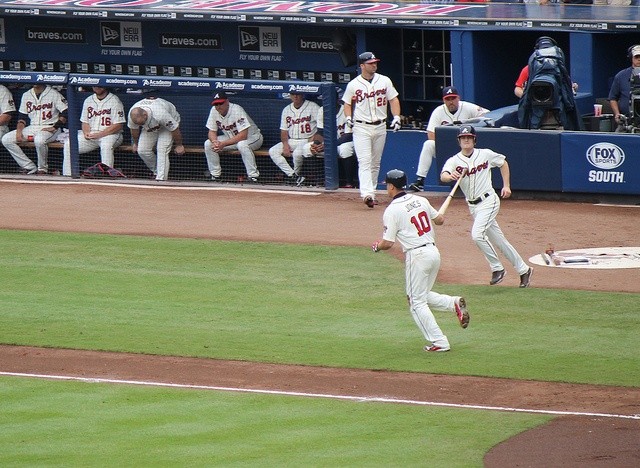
[518,45,576,130]
[619,67,640,133]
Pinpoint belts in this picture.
[465,192,489,203]
[356,118,388,125]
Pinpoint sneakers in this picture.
[454,296,469,327]
[409,181,424,191]
[519,266,533,286]
[490,268,507,284]
[423,340,450,352]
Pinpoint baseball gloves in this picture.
[371,240,380,253]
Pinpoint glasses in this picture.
[213,102,224,105]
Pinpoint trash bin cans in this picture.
[582,113,616,132]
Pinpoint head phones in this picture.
[626,44,639,67]
[532,36,558,54]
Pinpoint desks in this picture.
[435,91,639,194]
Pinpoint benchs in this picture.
[14,133,342,178]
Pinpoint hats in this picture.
[443,86,458,99]
[383,169,407,188]
[211,91,227,104]
[632,45,640,57]
[358,51,381,66]
[457,125,477,138]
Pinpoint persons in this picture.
[1,83,68,175]
[514,37,579,99]
[268,89,321,187]
[0,84,17,142]
[439,125,534,289]
[302,90,357,187]
[127,96,186,182]
[203,91,264,182]
[371,169,470,353]
[340,50,402,209]
[63,86,126,177]
[409,86,491,192]
[608,44,640,132]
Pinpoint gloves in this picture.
[390,115,401,132]
[347,115,354,128]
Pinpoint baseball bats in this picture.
[542,251,550,265]
[437,167,469,215]
[546,250,560,265]
